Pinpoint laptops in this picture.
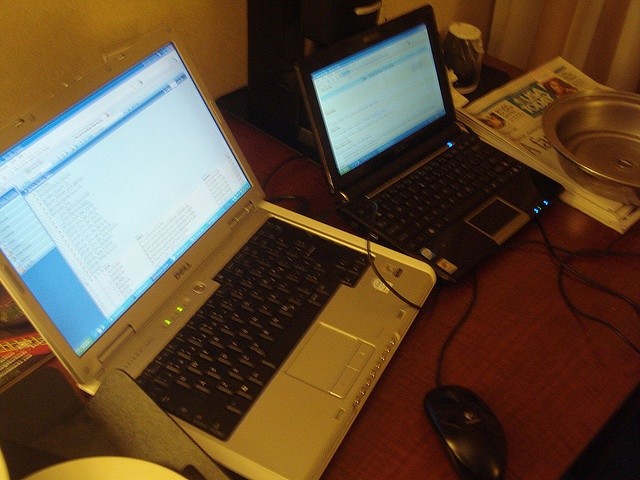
[1,26,437,480]
[294,5,564,282]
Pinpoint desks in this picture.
[0,47,639,478]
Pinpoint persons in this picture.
[547,77,579,100]
[481,113,506,129]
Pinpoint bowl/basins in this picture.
[542,91,640,206]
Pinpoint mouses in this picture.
[424,384,507,480]
